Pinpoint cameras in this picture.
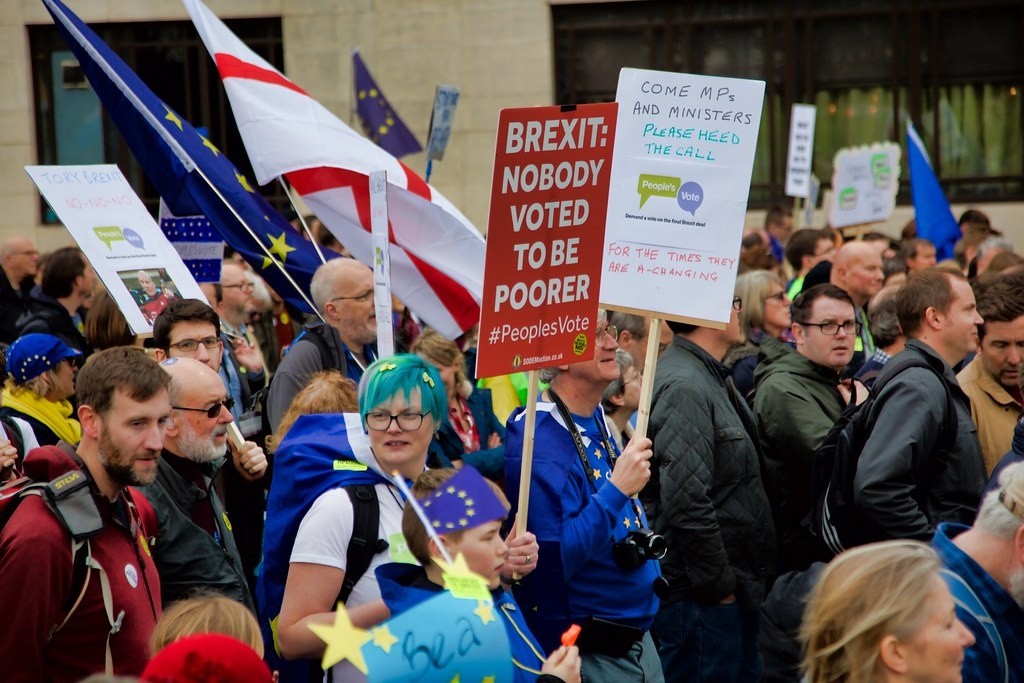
[612,528,669,571]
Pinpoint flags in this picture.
[183,0,486,342]
[41,0,398,326]
[905,119,963,262]
[353,52,423,159]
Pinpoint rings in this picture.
[526,555,530,564]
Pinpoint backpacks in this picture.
[256,330,336,489]
[800,359,958,554]
[757,562,1008,683]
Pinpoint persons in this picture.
[0,209,1024,683]
[136,270,180,327]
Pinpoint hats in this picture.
[960,210,1000,236]
[6,333,83,384]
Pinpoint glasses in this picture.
[7,250,39,258]
[170,337,222,351]
[64,357,76,366]
[595,325,617,347]
[733,295,741,310]
[365,409,432,431]
[221,282,254,292]
[331,288,373,304]
[172,398,234,418]
[764,291,785,302]
[799,320,860,335]
